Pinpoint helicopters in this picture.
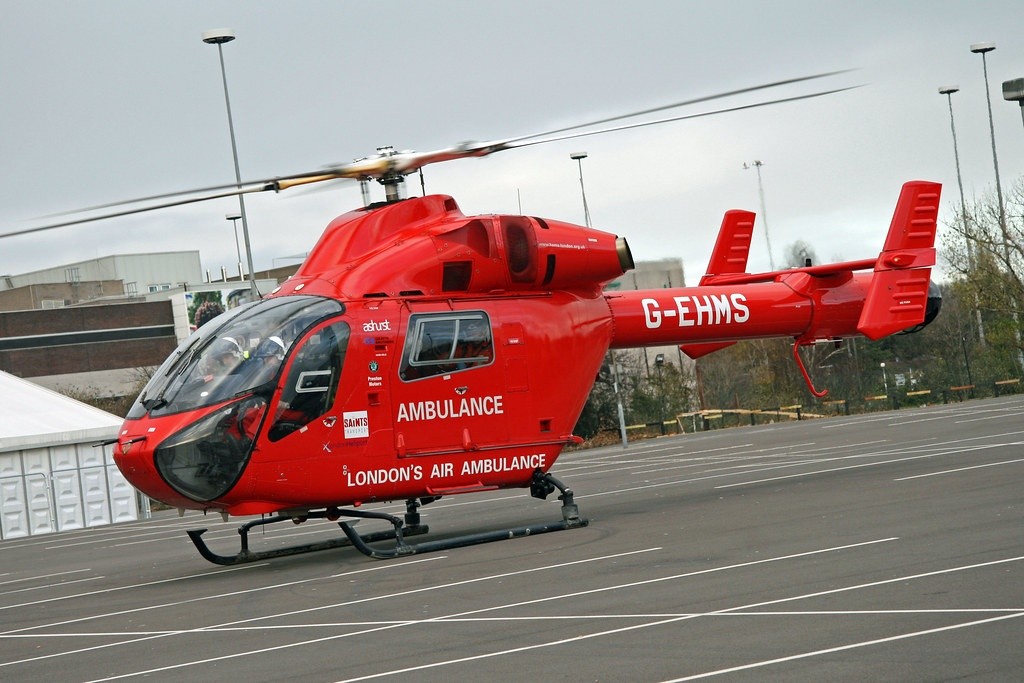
[1,64,943,566]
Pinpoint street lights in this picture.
[742,158,775,271]
[881,363,888,394]
[939,84,985,350]
[570,151,630,449]
[202,28,258,304]
[970,40,1024,369]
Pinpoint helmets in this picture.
[219,337,244,361]
[253,336,285,360]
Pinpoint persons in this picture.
[434,323,493,367]
[212,337,260,387]
[222,336,308,450]
